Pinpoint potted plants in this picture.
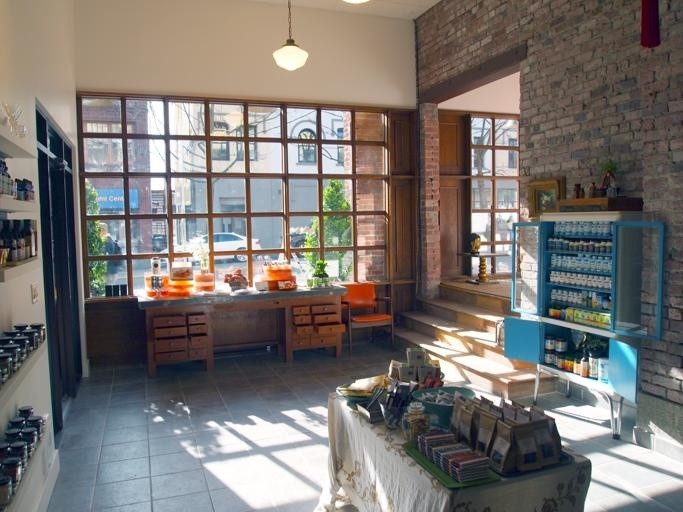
[306,260,330,288]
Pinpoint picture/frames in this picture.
[528,178,559,215]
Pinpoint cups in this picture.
[151,275,165,297]
[151,257,160,276]
[105,284,127,296]
[199,255,210,273]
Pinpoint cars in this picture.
[160,232,261,266]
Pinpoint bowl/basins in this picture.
[411,386,477,417]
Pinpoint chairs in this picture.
[342,282,394,360]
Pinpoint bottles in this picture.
[549,270,611,289]
[588,182,598,198]
[549,252,612,273]
[157,276,162,289]
[150,256,160,275]
[0,323,45,388]
[550,289,611,311]
[1,219,36,266]
[573,183,582,199]
[571,331,608,382]
[546,236,611,252]
[544,334,574,372]
[0,405,44,506]
[312,270,329,288]
[0,159,35,201]
[553,221,610,237]
[151,276,156,290]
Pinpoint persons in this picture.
[99,223,123,285]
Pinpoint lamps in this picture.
[274,2,308,71]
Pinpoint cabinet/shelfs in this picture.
[137,285,346,378]
[319,377,589,512]
[504,209,666,440]
[0,128,60,511]
